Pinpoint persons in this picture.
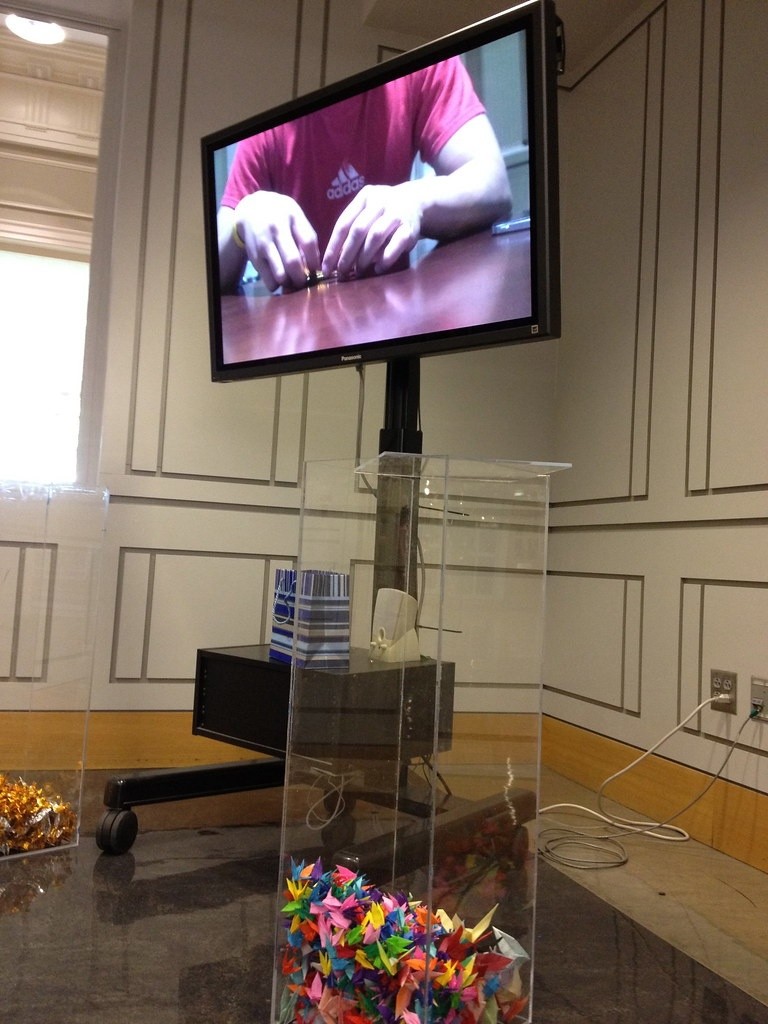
[217,56,514,296]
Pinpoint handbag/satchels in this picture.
[270,569,351,669]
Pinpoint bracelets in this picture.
[231,221,246,249]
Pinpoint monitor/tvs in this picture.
[198,0,564,385]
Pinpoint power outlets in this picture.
[710,668,737,715]
